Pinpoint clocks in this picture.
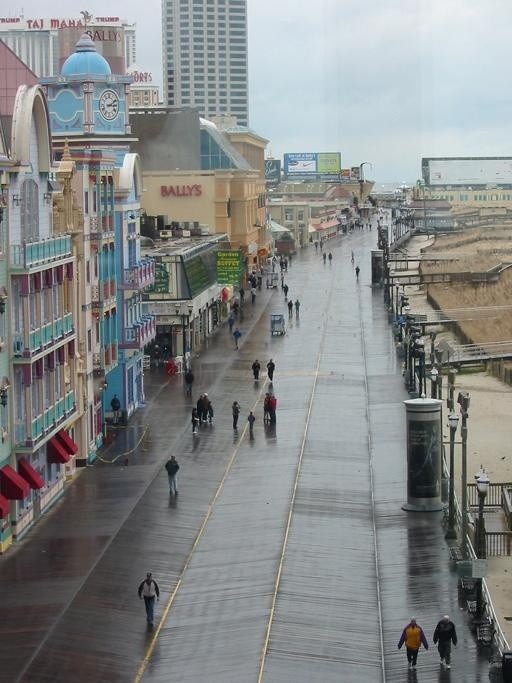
[99,89,120,122]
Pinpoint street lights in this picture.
[448,409,458,539]
[384,262,438,399]
[175,302,193,374]
[476,465,490,558]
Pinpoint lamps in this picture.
[0,389,7,408]
[0,296,7,314]
[104,382,107,390]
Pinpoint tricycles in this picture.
[270,314,285,335]
[267,271,279,288]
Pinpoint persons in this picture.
[281,272,300,318]
[184,358,278,435]
[239,268,262,305]
[433,615,457,669]
[355,266,360,280]
[271,256,292,273]
[358,217,383,231]
[397,616,428,671]
[111,393,121,425]
[227,301,241,351]
[314,239,331,265]
[164,455,180,494]
[154,345,162,367]
[138,572,160,627]
